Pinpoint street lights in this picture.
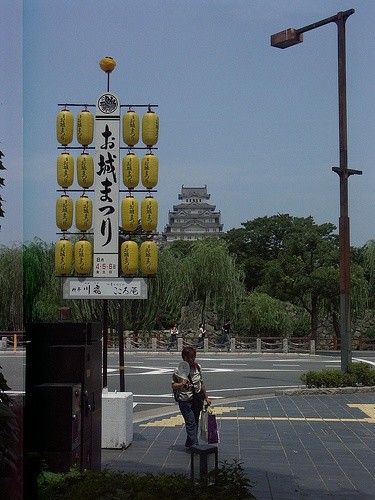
[270,8,364,370]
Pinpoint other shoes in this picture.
[185,442,198,448]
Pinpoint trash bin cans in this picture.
[100,390,134,449]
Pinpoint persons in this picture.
[170,323,178,349]
[218,319,232,352]
[171,347,211,448]
[197,323,206,349]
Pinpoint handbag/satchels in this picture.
[194,392,204,411]
[200,404,218,444]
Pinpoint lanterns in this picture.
[56,109,94,276]
[121,110,160,275]
